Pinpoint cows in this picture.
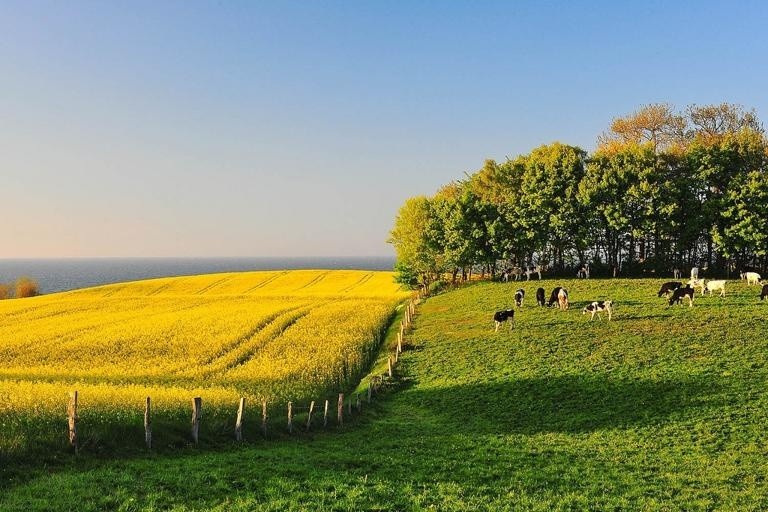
[536,287,545,307]
[657,278,727,308]
[739,269,747,281]
[747,272,764,287]
[493,308,515,332]
[690,267,698,280]
[514,288,525,309]
[575,263,590,279]
[500,266,542,283]
[547,286,568,310]
[756,284,768,301]
[673,266,681,279]
[582,300,612,322]
